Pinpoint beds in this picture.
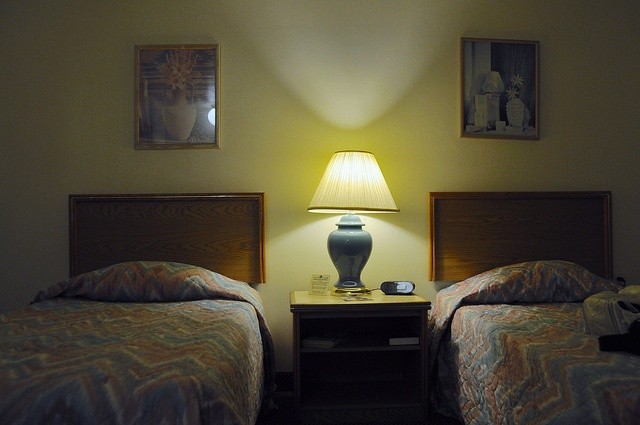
[427,190,639,425]
[1,193,277,425]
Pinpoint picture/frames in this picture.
[134,43,222,151]
[459,36,540,141]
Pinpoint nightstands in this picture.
[289,290,432,423]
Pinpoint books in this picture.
[388,336,421,348]
[300,337,340,350]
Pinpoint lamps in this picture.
[306,150,400,293]
